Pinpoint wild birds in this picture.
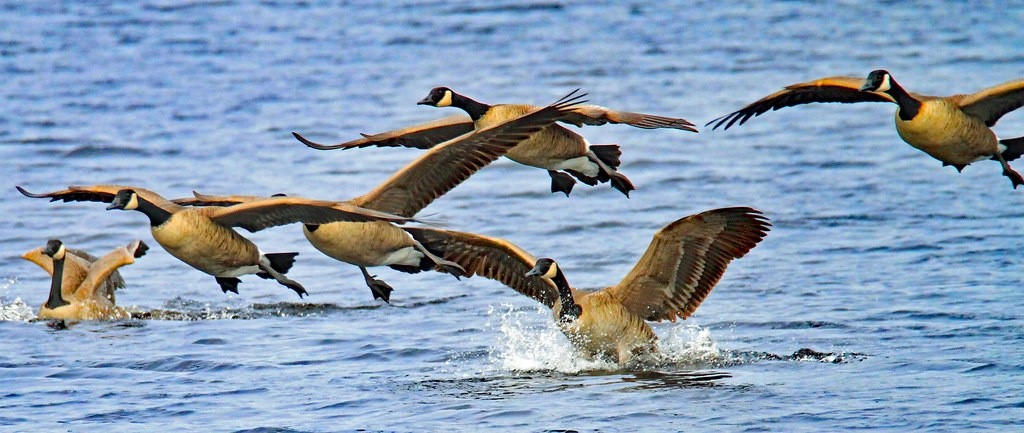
[13,183,449,300]
[703,69,1024,190]
[389,206,774,365]
[291,88,699,200]
[20,238,149,322]
[169,88,589,306]
